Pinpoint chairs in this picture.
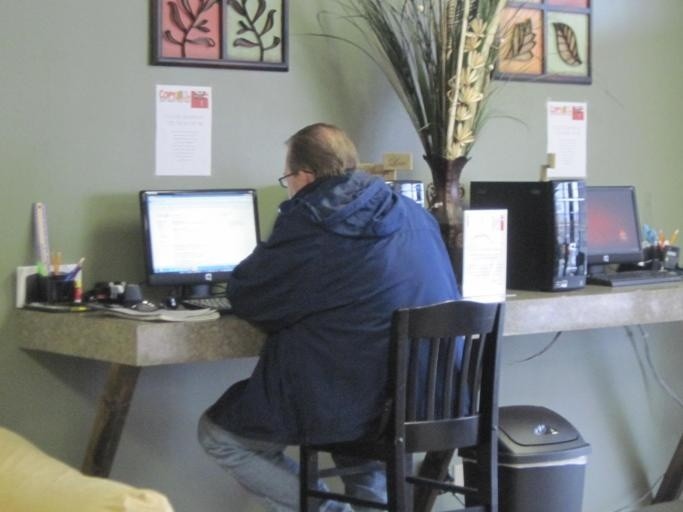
[298,300,507,512]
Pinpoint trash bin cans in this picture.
[458,405,593,512]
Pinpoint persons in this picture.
[197,123,472,512]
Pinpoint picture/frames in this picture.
[149,0,290,73]
[491,0,593,85]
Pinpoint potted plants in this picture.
[296,0,624,299]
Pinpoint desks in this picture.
[9,281,682,477]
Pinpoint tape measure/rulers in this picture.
[33,202,53,275]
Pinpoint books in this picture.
[102,307,220,321]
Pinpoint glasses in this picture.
[279,171,313,189]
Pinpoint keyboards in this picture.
[181,297,231,315]
[587,269,683,288]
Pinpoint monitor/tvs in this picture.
[587,186,644,274]
[140,189,260,299]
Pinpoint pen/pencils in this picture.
[37,252,85,282]
[642,224,680,247]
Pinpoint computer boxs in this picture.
[469,178,587,291]
[384,179,425,208]
